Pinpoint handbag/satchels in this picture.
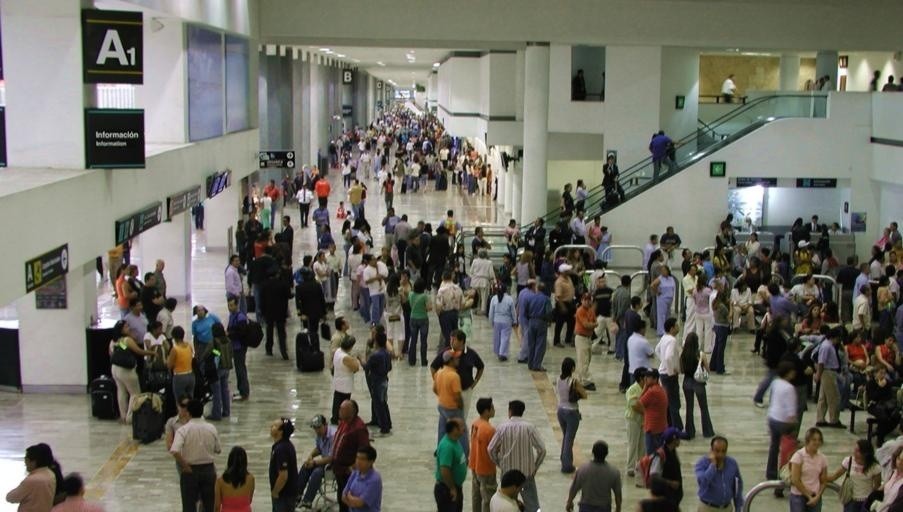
[779,451,803,489]
[388,314,401,323]
[236,321,264,348]
[320,322,332,342]
[568,379,582,403]
[838,456,854,506]
[692,351,709,385]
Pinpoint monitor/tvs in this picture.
[208,176,220,197]
[217,175,228,193]
[501,152,508,172]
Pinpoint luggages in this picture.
[88,374,120,422]
[132,395,166,444]
[294,329,325,373]
[139,366,177,418]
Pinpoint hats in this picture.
[580,292,591,301]
[796,239,810,248]
[558,263,573,272]
[639,367,660,380]
[660,427,690,443]
[309,414,326,429]
[442,349,463,364]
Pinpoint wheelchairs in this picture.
[295,451,340,512]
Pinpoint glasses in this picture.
[179,402,189,409]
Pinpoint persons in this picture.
[571,69,587,100]
[52,471,106,512]
[721,74,741,104]
[38,443,66,506]
[7,446,56,512]
[868,70,903,92]
[805,74,834,92]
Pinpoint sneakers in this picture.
[498,354,508,362]
[207,414,222,421]
[372,428,393,438]
[537,366,548,371]
[295,500,312,512]
[583,382,597,391]
[232,390,248,401]
[816,421,847,429]
